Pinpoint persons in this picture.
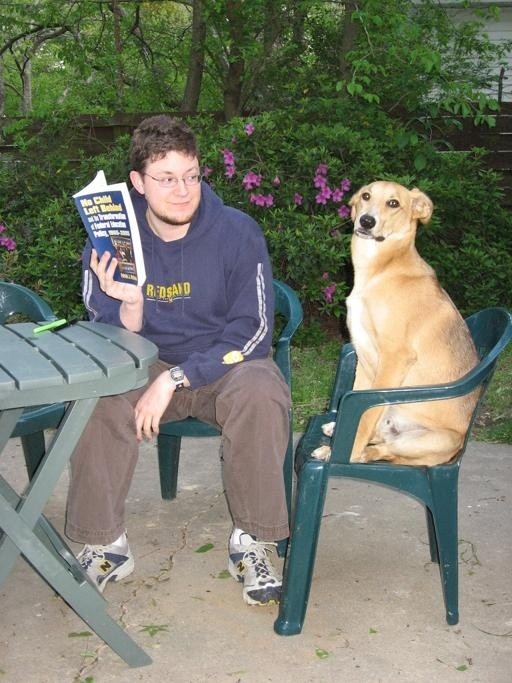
[64,112,294,609]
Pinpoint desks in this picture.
[0,319,159,666]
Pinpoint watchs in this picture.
[167,364,186,393]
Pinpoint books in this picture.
[72,168,147,287]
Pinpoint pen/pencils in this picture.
[33,318,67,334]
[52,316,78,332]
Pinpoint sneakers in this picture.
[227,528,282,606]
[75,528,134,594]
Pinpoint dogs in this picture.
[309,180,484,469]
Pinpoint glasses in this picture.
[143,173,202,187]
